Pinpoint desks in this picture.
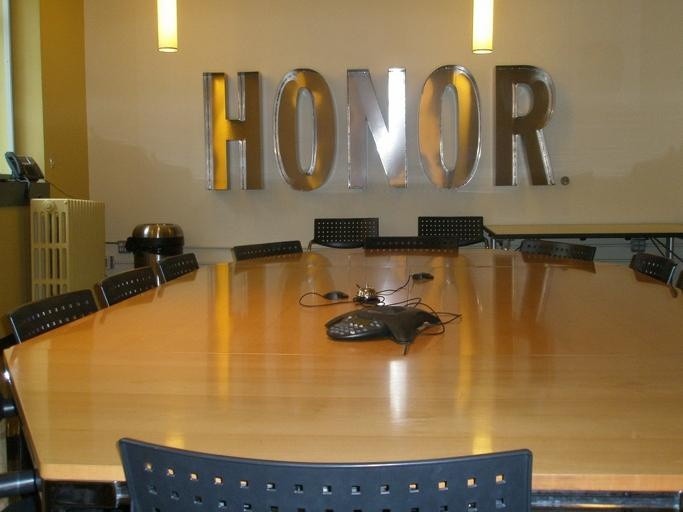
[7,256,682,493]
[483,217,683,254]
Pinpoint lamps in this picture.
[471,0,494,55]
[156,0,178,53]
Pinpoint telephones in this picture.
[325,306,441,343]
[5,152,44,180]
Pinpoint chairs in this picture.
[99,265,158,306]
[629,251,678,284]
[114,433,534,510]
[309,217,380,248]
[230,240,303,261]
[519,239,596,263]
[9,288,100,342]
[157,252,199,281]
[362,234,459,253]
[417,217,490,249]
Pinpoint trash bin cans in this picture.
[125,223,184,267]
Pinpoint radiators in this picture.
[29,198,105,302]
